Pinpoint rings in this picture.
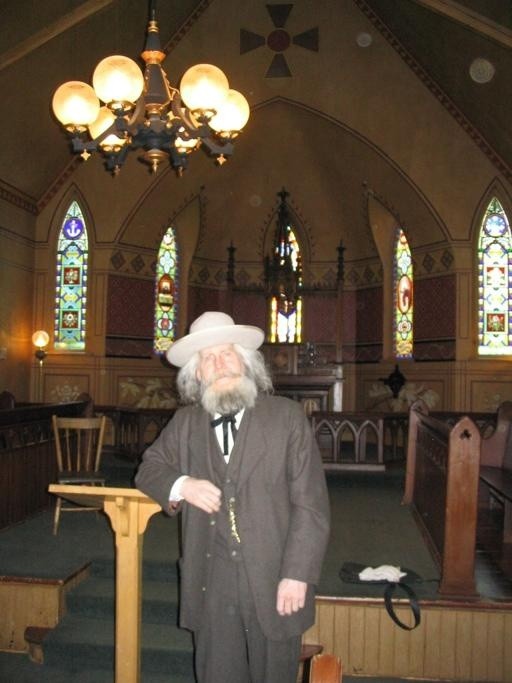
[292,599,298,603]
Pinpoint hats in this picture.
[166,311,265,367]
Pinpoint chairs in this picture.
[49,413,107,535]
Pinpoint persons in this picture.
[134,311,331,682]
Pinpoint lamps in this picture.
[31,328,49,359]
[51,0,250,177]
